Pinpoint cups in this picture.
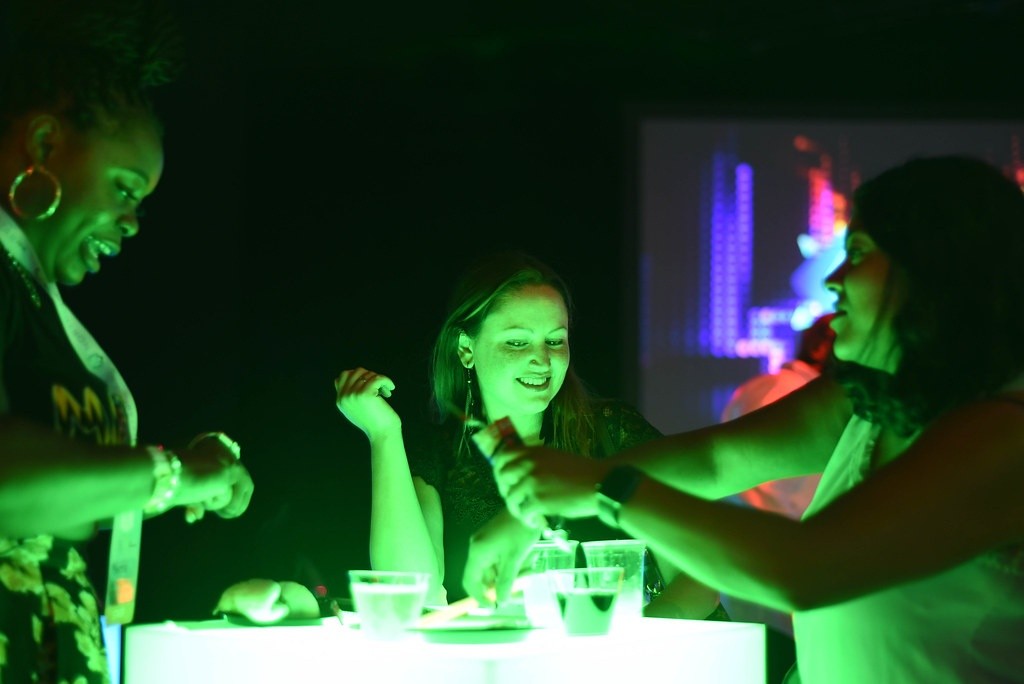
[530,538,646,636]
[348,569,428,628]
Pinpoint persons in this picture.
[0,0,254,684]
[714,313,835,684]
[332,245,718,622]
[494,155,1023,684]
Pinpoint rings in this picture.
[359,376,367,384]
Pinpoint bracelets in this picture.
[142,445,181,516]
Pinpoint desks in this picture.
[122,616,768,684]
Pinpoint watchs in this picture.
[596,463,641,528]
